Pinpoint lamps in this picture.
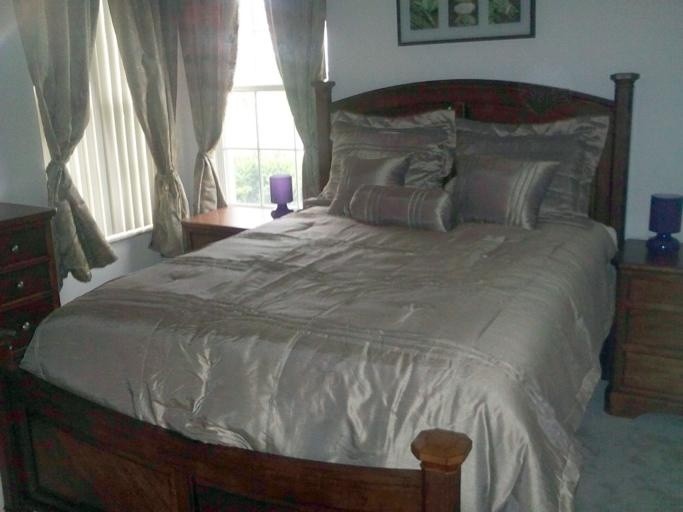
[270,175,294,219]
[645,193,683,254]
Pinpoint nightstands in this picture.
[607,238,683,420]
[181,204,277,253]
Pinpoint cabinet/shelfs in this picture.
[0,202,62,388]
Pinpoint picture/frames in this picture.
[397,0,536,46]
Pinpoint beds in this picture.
[15,72,640,512]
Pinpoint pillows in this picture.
[445,162,559,230]
[349,184,452,232]
[454,115,609,227]
[328,155,409,217]
[304,106,459,206]
